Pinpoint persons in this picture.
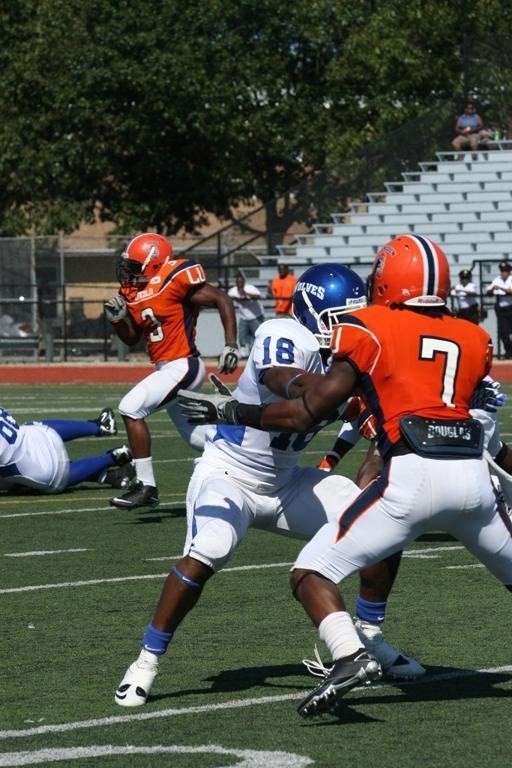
[0,406,136,498]
[102,234,239,512]
[452,103,483,151]
[114,233,512,720]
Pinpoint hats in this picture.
[499,262,511,272]
[458,270,472,279]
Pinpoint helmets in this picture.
[292,263,367,349]
[114,231,174,289]
[373,234,451,306]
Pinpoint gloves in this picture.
[104,297,128,324]
[176,372,238,424]
[217,344,238,374]
[470,381,505,412]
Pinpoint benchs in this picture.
[217,137,512,311]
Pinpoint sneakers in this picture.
[109,445,158,512]
[298,647,383,718]
[88,408,116,434]
[363,641,428,679]
[114,649,159,707]
